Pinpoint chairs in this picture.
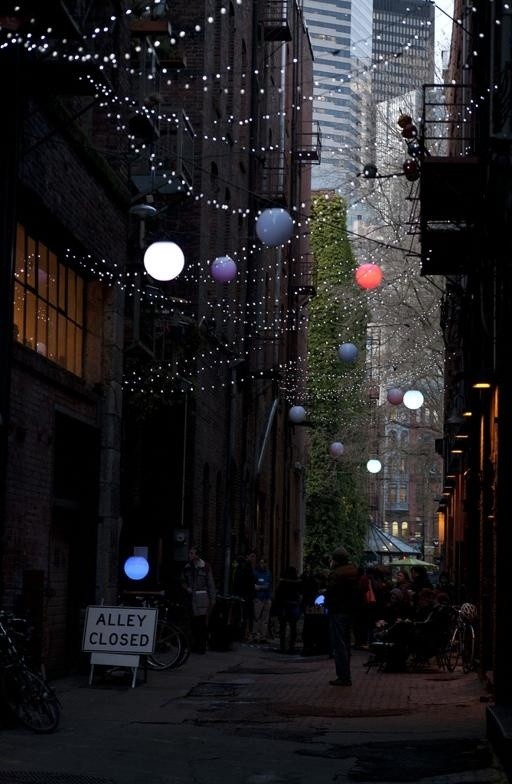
[363,605,459,673]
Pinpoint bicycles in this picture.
[85,588,192,674]
[0,610,62,733]
[438,603,476,673]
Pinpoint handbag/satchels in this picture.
[365,580,376,605]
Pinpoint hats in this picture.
[390,588,401,598]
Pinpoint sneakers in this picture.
[248,639,269,644]
[329,679,352,686]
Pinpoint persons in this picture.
[233,550,453,673]
[179,545,217,655]
[323,547,354,687]
